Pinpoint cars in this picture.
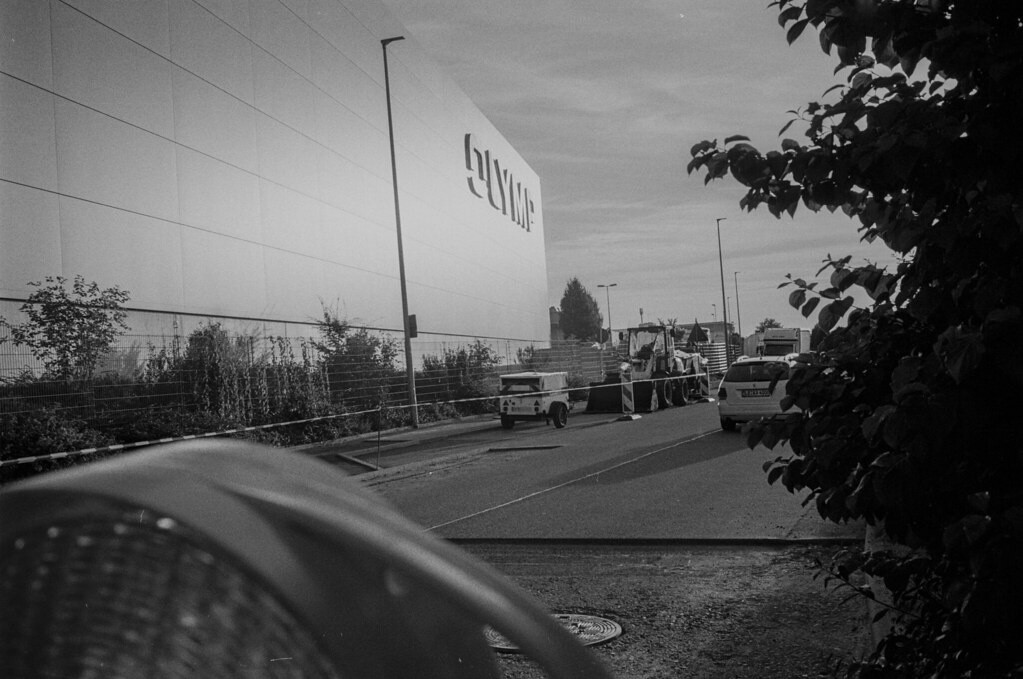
[496,370,572,428]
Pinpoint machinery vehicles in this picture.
[583,324,703,415]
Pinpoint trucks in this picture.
[756,327,811,356]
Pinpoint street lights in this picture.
[717,217,730,370]
[596,282,618,347]
[727,296,732,345]
[380,35,420,432]
[735,271,742,345]
[712,303,717,323]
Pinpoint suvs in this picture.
[717,351,810,432]
